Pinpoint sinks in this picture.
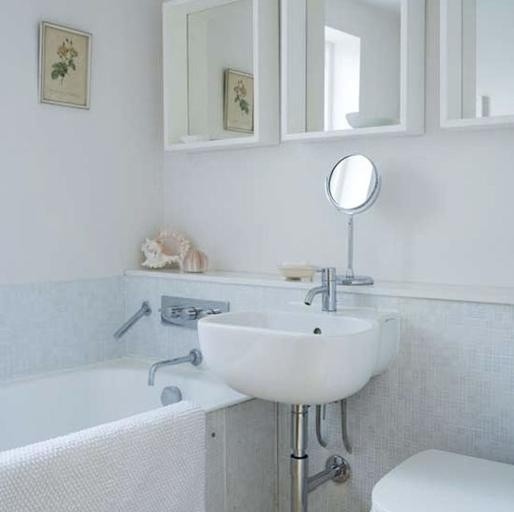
[198,309,378,404]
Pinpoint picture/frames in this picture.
[223,68,255,135]
[37,20,93,110]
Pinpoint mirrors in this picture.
[281,0,426,143]
[325,153,381,285]
[438,0,514,131]
[162,0,281,152]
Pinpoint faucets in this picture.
[148,348,202,387]
[304,266,337,312]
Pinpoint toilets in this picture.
[372,446,514,510]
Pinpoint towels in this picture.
[0,400,205,512]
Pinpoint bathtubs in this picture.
[0,354,295,453]
